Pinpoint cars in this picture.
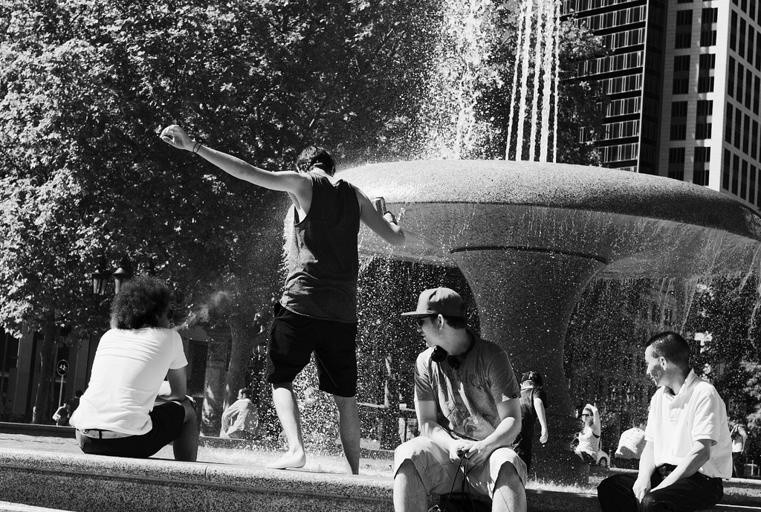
[594,449,611,470]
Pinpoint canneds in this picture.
[373,196,386,216]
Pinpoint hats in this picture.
[401,287,467,317]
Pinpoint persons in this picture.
[219,386,260,439]
[52,398,71,425]
[69,389,83,414]
[574,398,604,465]
[726,415,761,478]
[391,286,530,512]
[613,412,648,468]
[63,272,200,461]
[514,370,549,474]
[597,331,733,512]
[160,124,407,475]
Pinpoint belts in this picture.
[76,429,134,439]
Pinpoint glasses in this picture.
[582,414,589,417]
[417,315,443,324]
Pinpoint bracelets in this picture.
[387,218,397,223]
[194,139,201,154]
